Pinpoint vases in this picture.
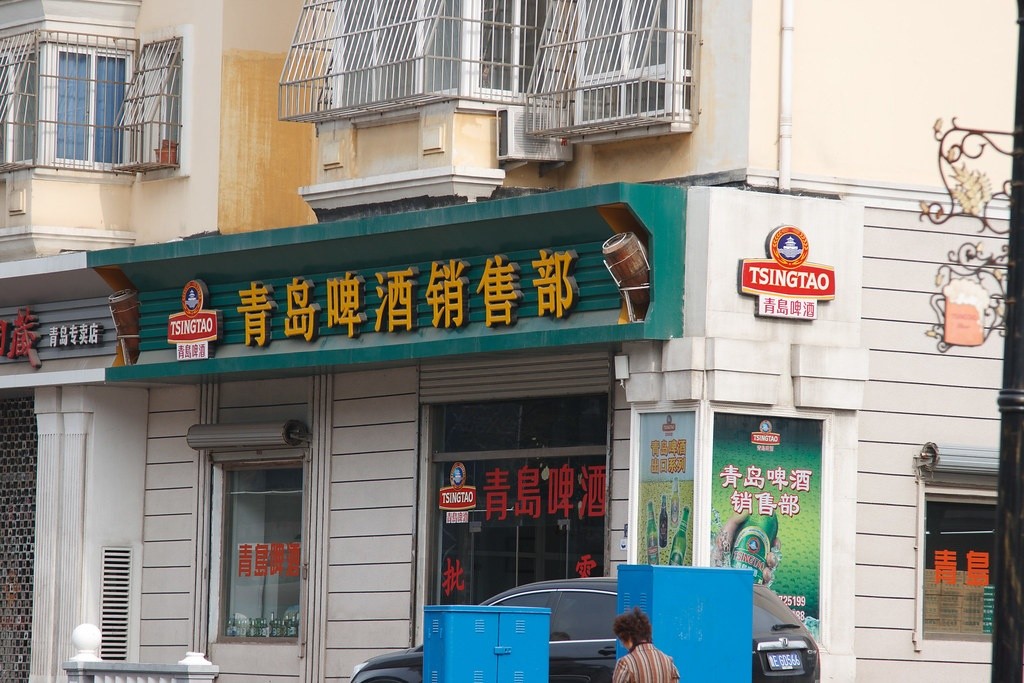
[154,139,178,165]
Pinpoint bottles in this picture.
[646,501,659,566]
[669,508,689,566]
[670,476,680,528]
[226,612,298,637]
[658,496,668,547]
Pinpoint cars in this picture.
[350,577,820,683]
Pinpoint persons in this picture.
[612,605,680,683]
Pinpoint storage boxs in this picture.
[925,568,994,637]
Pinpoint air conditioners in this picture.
[496,105,573,163]
